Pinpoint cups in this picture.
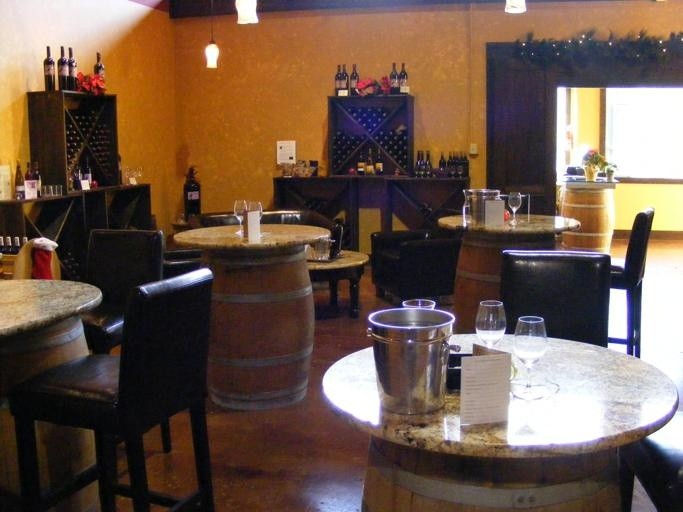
[40,185,46,196]
[249,202,262,226]
[47,186,53,195]
[404,298,435,310]
[53,185,57,196]
[514,316,544,399]
[234,199,246,236]
[474,301,505,349]
[507,193,522,227]
[56,185,62,195]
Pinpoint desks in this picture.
[561,174,620,254]
[173,224,331,410]
[438,214,581,334]
[381,177,470,232]
[0,195,78,260]
[304,249,370,319]
[322,333,680,512]
[273,177,359,253]
[0,279,103,512]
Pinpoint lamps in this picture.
[504,0,526,14]
[204,0,219,68]
[234,0,259,24]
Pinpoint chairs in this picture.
[78,229,164,355]
[7,267,215,512]
[499,249,611,348]
[608,208,655,358]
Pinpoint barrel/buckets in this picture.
[361,434,623,511]
[1,315,101,512]
[203,250,317,410]
[558,188,617,256]
[367,308,456,416]
[453,236,558,330]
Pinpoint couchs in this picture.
[370,208,466,309]
[163,249,203,280]
[199,209,344,252]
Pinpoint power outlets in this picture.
[277,141,296,165]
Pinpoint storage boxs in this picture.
[27,90,121,195]
[327,95,414,177]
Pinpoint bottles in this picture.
[83,155,92,187]
[43,46,56,91]
[350,64,359,96]
[415,150,432,177]
[0,237,4,254]
[32,161,42,198]
[398,63,408,94]
[25,162,32,180]
[4,237,13,254]
[439,152,446,168]
[335,65,341,96]
[68,47,77,91]
[13,237,20,254]
[366,148,374,175]
[447,152,469,177]
[15,159,25,198]
[340,64,349,90]
[57,46,69,91]
[390,63,398,94]
[357,151,366,175]
[94,52,105,77]
[375,149,383,174]
